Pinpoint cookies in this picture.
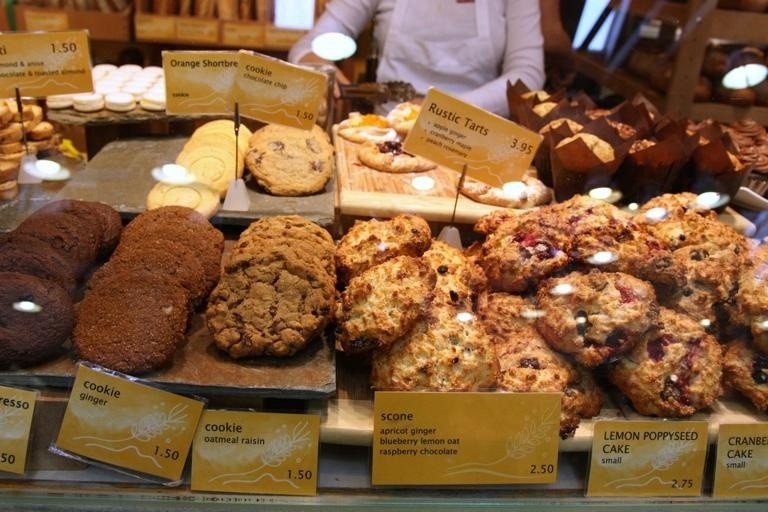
[0,118,767,438]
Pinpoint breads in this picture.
[0,64,166,201]
[505,75,768,193]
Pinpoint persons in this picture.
[288,0,546,117]
